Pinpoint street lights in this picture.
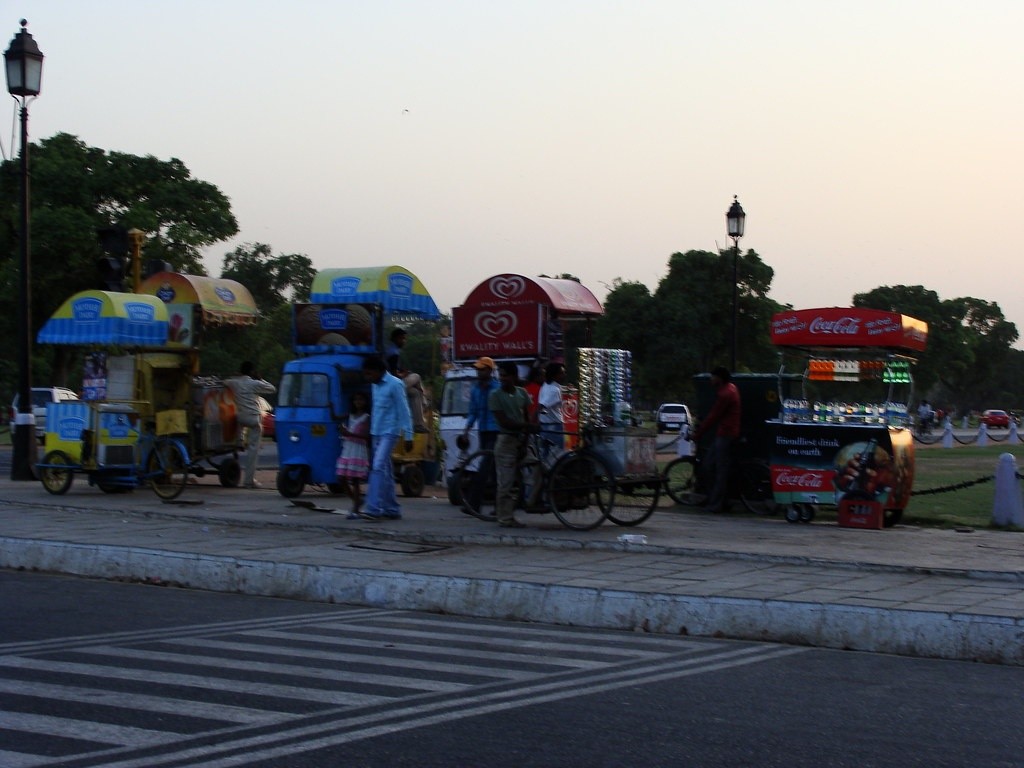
[2,22,45,481]
[726,196,746,371]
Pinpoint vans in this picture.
[656,404,692,433]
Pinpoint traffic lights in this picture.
[94,227,131,280]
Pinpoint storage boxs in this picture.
[593,426,655,478]
[839,500,883,529]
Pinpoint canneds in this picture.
[169,313,191,341]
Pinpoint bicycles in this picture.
[915,417,932,436]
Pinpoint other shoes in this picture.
[708,504,722,512]
[414,425,430,434]
[245,481,262,489]
[499,521,526,528]
[460,507,469,514]
[358,512,381,519]
[387,513,402,519]
[347,512,358,519]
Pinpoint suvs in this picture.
[10,386,79,445]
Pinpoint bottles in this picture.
[782,399,908,427]
[809,360,910,383]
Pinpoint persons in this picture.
[537,363,563,448]
[690,364,740,513]
[460,356,503,516]
[385,328,409,378]
[222,359,277,487]
[917,400,933,436]
[355,352,414,520]
[487,362,533,528]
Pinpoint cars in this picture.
[979,410,1009,428]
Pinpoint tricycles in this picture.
[454,409,661,531]
[664,306,927,527]
[35,272,263,499]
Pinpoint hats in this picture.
[474,357,494,369]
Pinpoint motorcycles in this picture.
[276,265,443,499]
[440,274,605,506]
